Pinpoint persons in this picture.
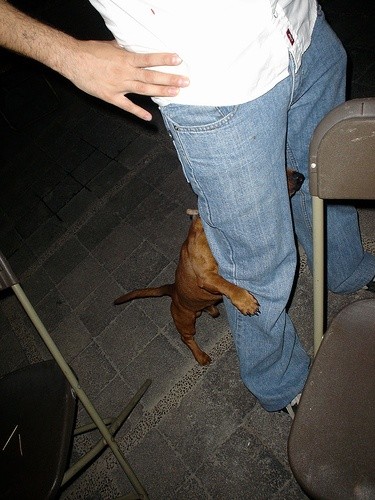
[0,0,375,422]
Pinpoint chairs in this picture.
[0,253,152,500]
[288,99,375,500]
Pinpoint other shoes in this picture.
[280,393,303,421]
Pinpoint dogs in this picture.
[113,169,306,365]
